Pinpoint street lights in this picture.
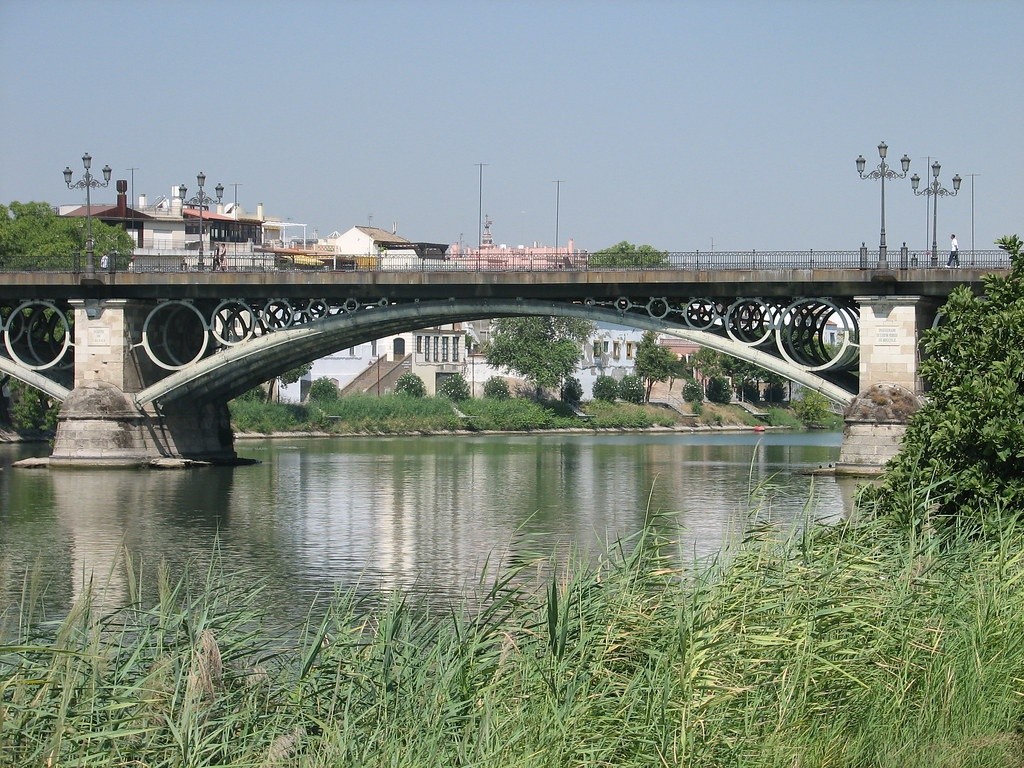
[63,152,112,272]
[179,172,226,272]
[856,140,911,270]
[914,160,963,267]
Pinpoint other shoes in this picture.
[955,265,961,268]
[945,264,951,268]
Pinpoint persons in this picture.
[218,243,229,272]
[213,245,220,272]
[100,253,109,270]
[946,234,962,268]
